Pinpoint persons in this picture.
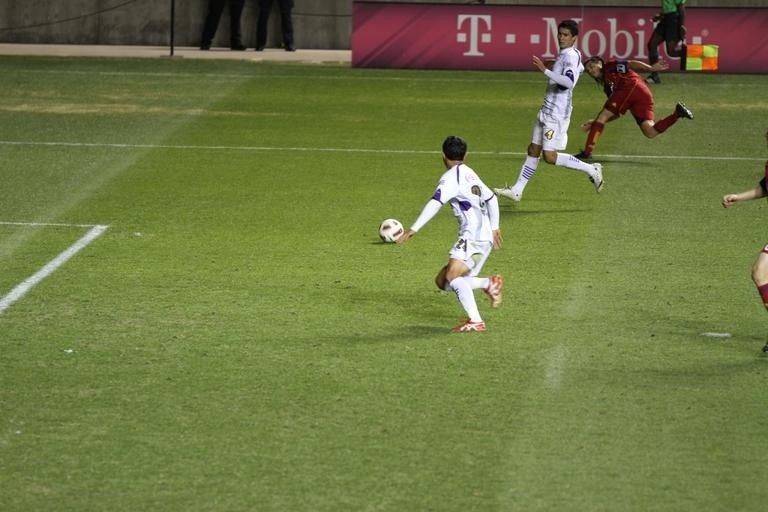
[641,0,688,86]
[396,133,505,333]
[199,0,247,52]
[490,20,605,202]
[571,53,693,160]
[719,158,768,354]
[253,0,298,52]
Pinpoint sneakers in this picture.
[591,163,603,193]
[676,101,693,119]
[644,76,662,84]
[493,187,521,202]
[484,275,504,310]
[451,319,486,331]
[573,152,591,158]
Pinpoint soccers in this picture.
[378,219,404,243]
